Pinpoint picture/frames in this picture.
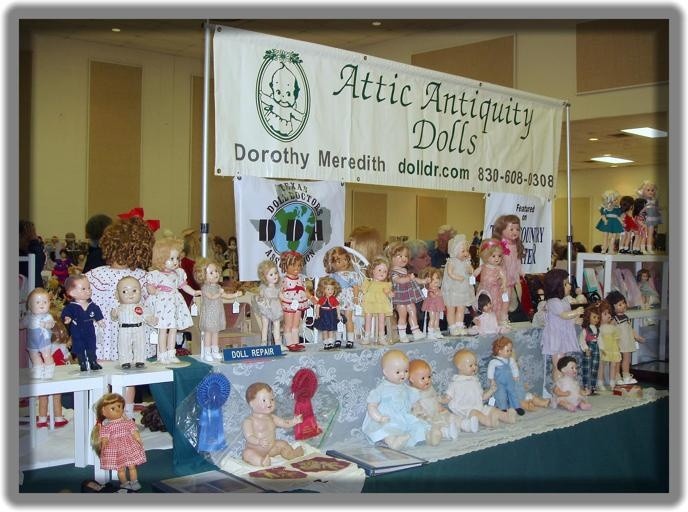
[20,363,109,485]
[19,367,668,493]
[99,359,174,481]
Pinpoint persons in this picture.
[86,210,201,369]
[180,228,256,361]
[444,349,516,427]
[64,275,104,372]
[553,357,592,411]
[639,269,659,310]
[38,323,70,428]
[362,350,442,449]
[252,214,522,354]
[409,359,479,439]
[533,268,646,395]
[514,383,551,411]
[20,216,113,300]
[488,337,525,416]
[91,393,147,490]
[243,382,303,466]
[21,287,56,378]
[596,182,662,255]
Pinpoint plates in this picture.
[576,253,669,366]
